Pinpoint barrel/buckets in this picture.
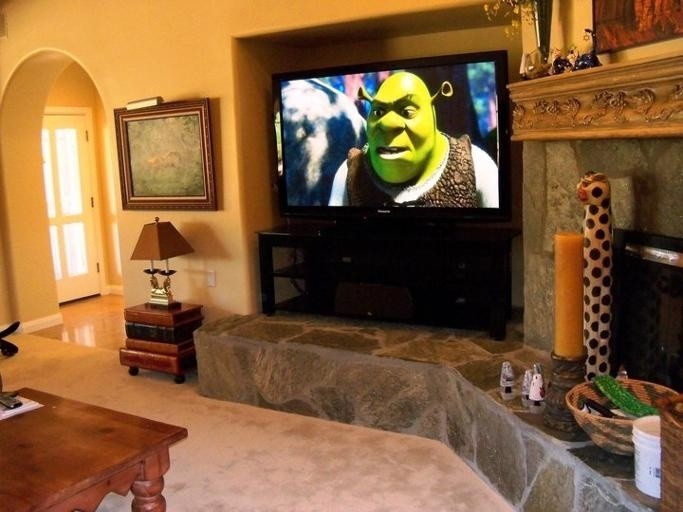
[630,413,663,500]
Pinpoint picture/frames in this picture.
[113,97,217,212]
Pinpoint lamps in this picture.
[129,216,195,310]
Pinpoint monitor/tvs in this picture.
[272,51,512,240]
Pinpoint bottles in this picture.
[499,358,548,415]
